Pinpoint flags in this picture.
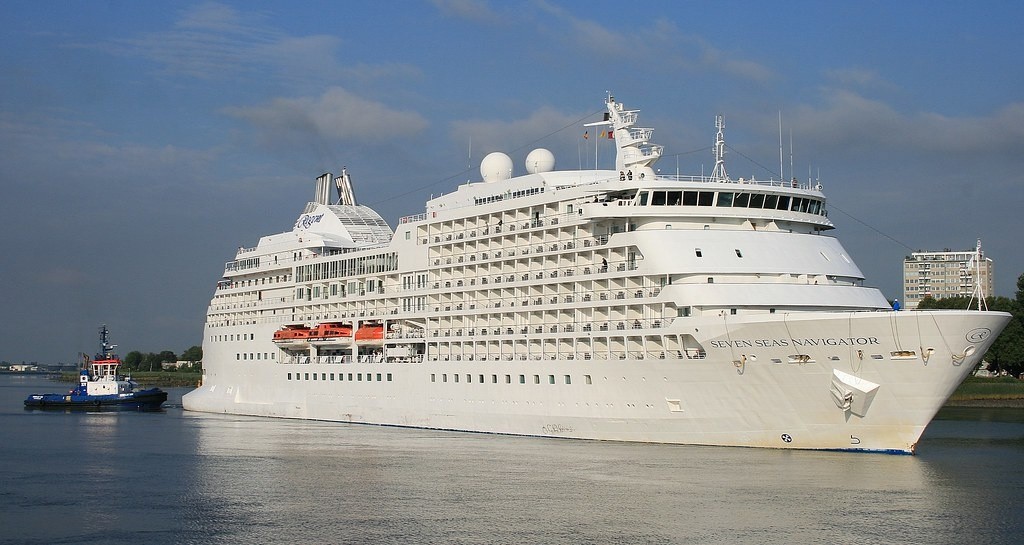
[584,131,588,139]
[599,130,613,140]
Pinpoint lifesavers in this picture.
[94,399,100,406]
[77,390,81,395]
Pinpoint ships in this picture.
[181,90,1012,455]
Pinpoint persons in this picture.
[892,299,900,311]
[792,177,797,188]
[483,222,489,235]
[620,170,632,181]
[634,319,641,324]
[602,258,608,272]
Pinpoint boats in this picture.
[23,325,169,411]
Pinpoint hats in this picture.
[895,299,898,301]
